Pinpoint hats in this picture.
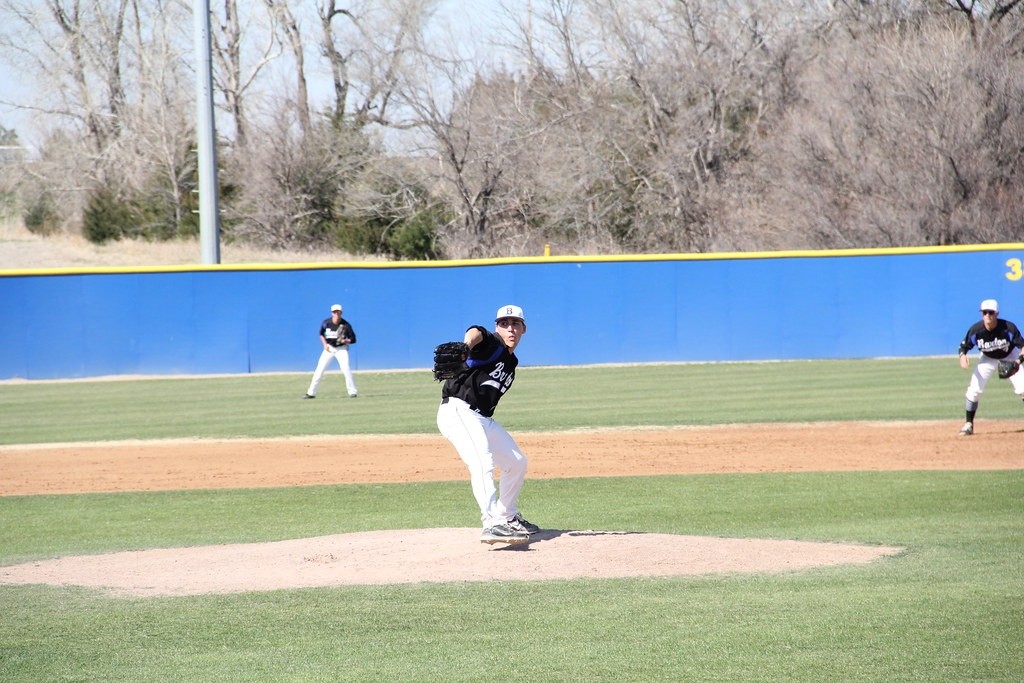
[979,300,999,313]
[495,305,526,335]
[331,304,342,312]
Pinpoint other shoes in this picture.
[351,394,357,397]
[302,394,315,399]
[959,423,973,436]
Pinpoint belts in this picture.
[442,395,484,416]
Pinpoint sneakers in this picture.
[507,512,539,534]
[480,523,529,545]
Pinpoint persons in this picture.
[302,304,357,398]
[434,304,539,543]
[959,299,1024,434]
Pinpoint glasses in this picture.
[982,310,998,315]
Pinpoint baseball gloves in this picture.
[997,359,1019,379]
[433,341,471,381]
[336,324,347,338]
